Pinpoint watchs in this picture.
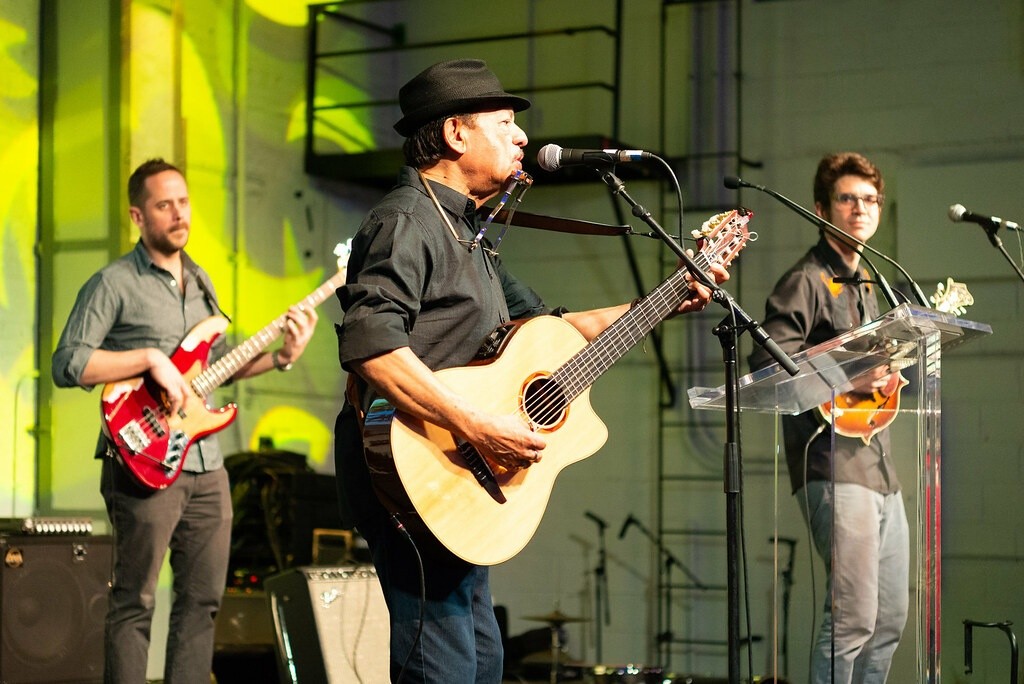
[273,350,293,372]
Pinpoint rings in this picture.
[531,449,537,460]
[881,377,884,381]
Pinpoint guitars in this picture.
[809,274,975,446]
[100,238,354,488]
[360,205,758,567]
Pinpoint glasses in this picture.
[829,192,883,206]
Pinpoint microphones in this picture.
[724,174,931,313]
[619,516,634,540]
[537,143,651,172]
[584,511,610,529]
[948,204,1022,232]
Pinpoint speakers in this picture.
[263,564,392,684]
[0,532,115,684]
[214,586,275,654]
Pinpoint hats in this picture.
[393,59,531,138]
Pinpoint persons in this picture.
[747,153,911,684]
[52,161,317,684]
[334,62,730,684]
